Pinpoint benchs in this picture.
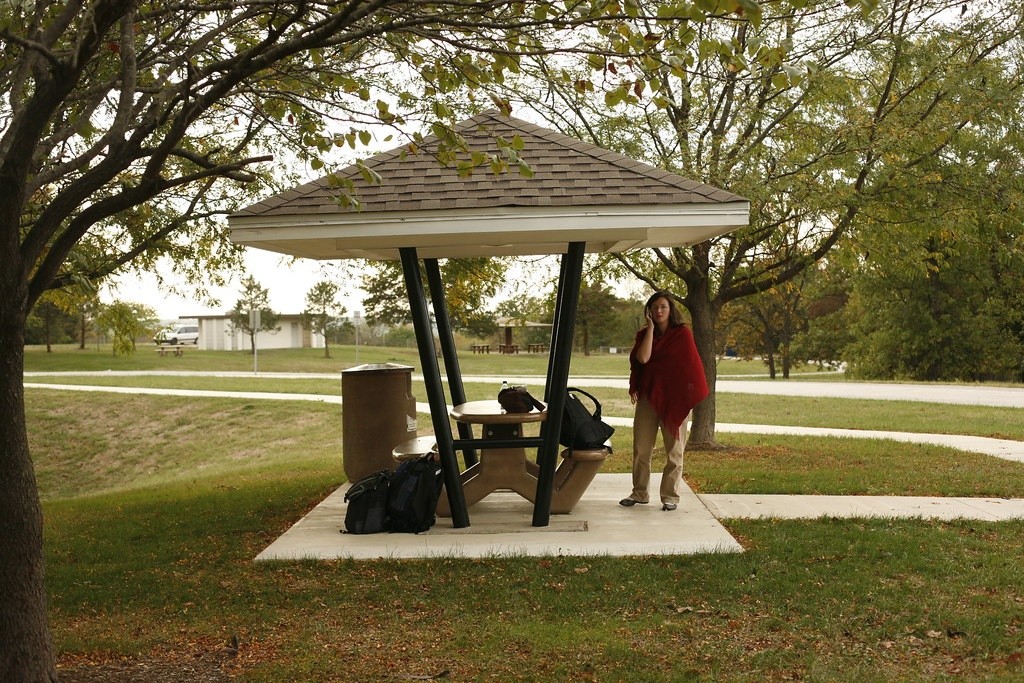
[496,348,522,350]
[391,435,454,518]
[470,348,492,350]
[525,347,548,349]
[554,439,613,513]
[155,349,183,354]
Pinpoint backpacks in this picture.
[340,451,443,534]
[559,387,614,450]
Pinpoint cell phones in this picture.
[650,312,652,318]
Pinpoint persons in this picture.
[619,291,710,510]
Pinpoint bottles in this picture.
[500,380,510,414]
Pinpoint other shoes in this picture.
[619,498,648,506]
[664,502,677,510]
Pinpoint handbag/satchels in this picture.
[497,386,545,413]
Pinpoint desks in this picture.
[498,344,521,354]
[158,345,182,356]
[471,345,491,354]
[452,399,548,507]
[527,343,547,354]
